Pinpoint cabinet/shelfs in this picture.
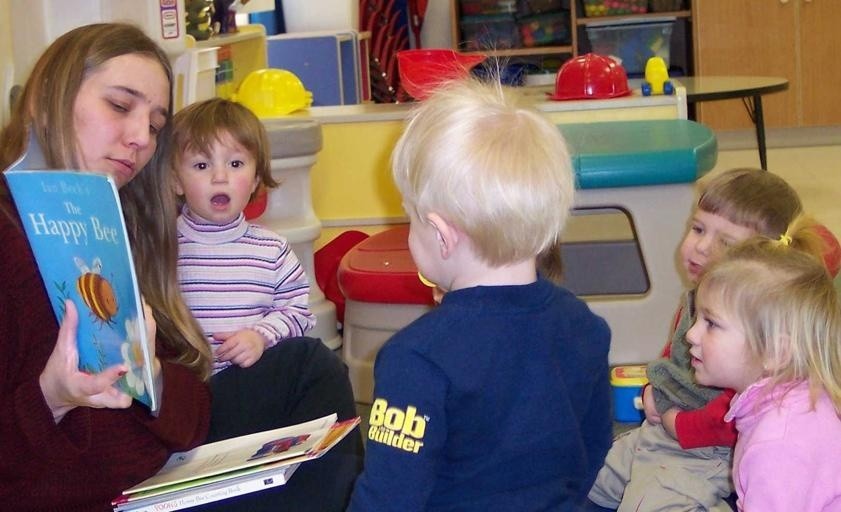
[448,0,841,132]
[266,31,372,106]
[187,23,267,111]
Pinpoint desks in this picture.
[622,72,790,173]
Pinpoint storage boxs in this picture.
[549,121,718,364]
[337,224,440,402]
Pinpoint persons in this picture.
[346,81,612,511]
[1,24,364,512]
[585,168,841,510]
